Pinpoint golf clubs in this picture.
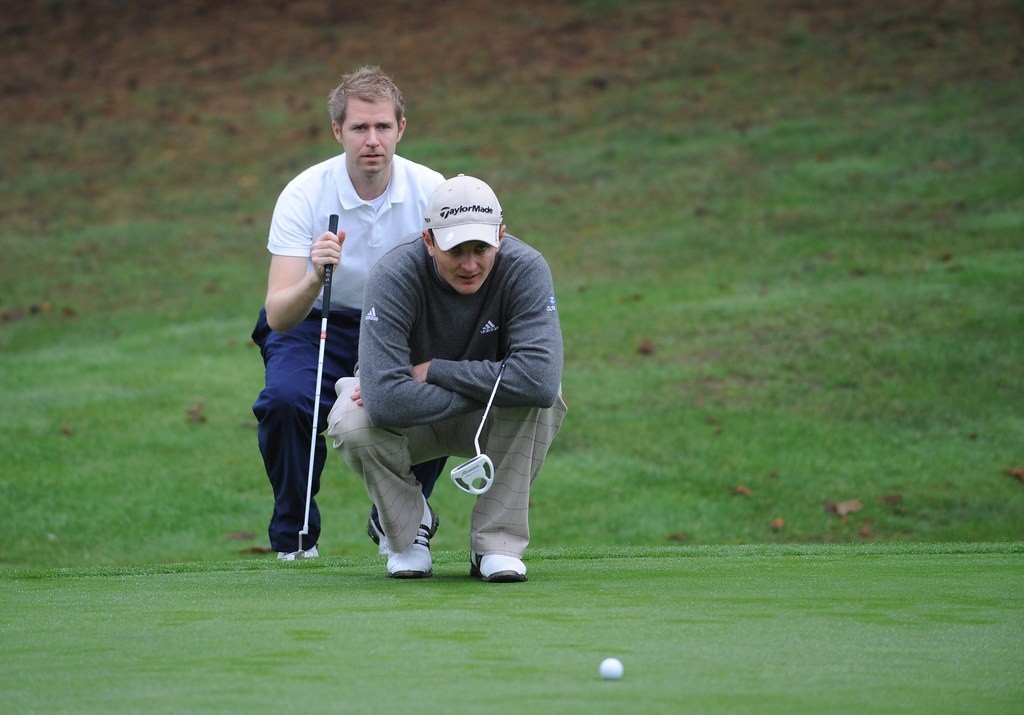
[449,346,509,497]
[293,213,340,558]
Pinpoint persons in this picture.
[326,174,568,584]
[249,66,450,561]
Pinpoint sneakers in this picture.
[277,544,319,560]
[367,515,387,554]
[469,548,529,582]
[387,493,439,578]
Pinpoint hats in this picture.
[425,172,504,250]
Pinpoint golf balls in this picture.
[598,657,624,681]
